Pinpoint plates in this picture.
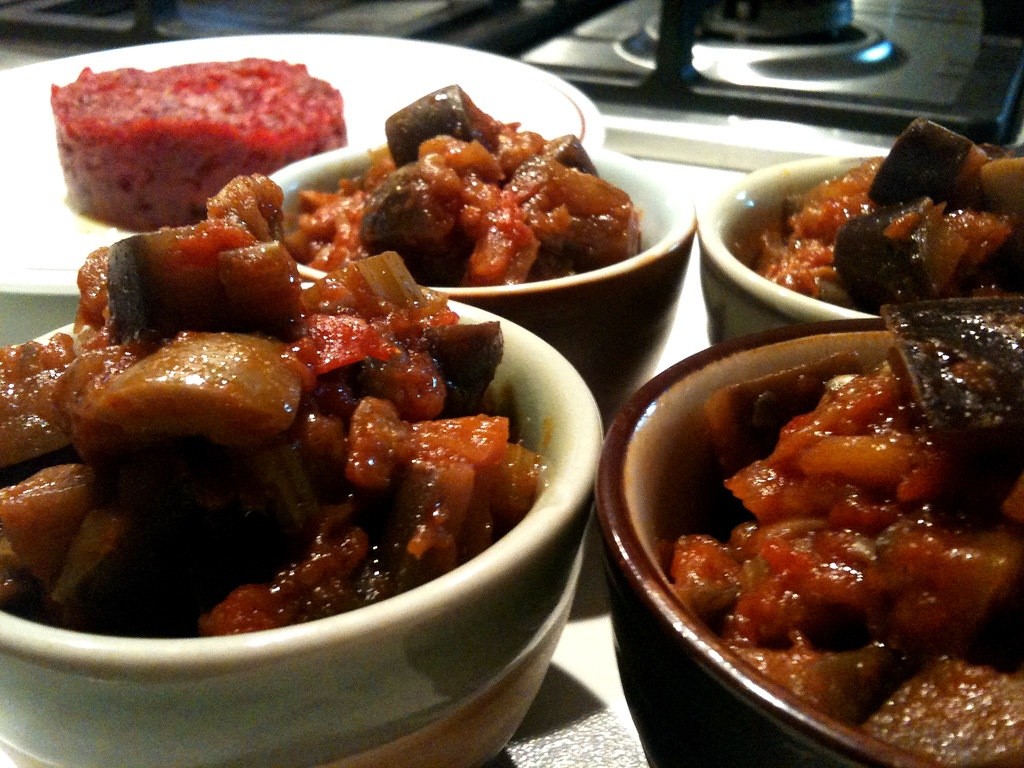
[0,32,606,294]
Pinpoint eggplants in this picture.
[0,84,1024,768]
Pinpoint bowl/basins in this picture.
[1,281,603,768]
[232,138,698,419]
[599,317,1023,768]
[694,151,908,355]
